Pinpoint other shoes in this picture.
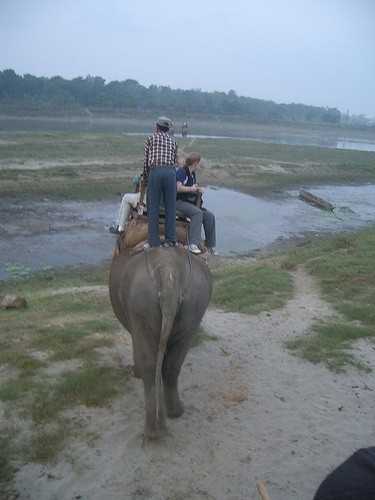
[164,240,178,248]
[144,243,162,249]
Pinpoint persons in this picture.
[108,116,219,257]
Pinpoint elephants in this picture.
[182,128,189,138]
[167,127,175,137]
[109,186,214,442]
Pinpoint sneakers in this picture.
[109,225,125,235]
[204,246,218,256]
[184,244,201,253]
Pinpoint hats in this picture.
[156,119,170,128]
